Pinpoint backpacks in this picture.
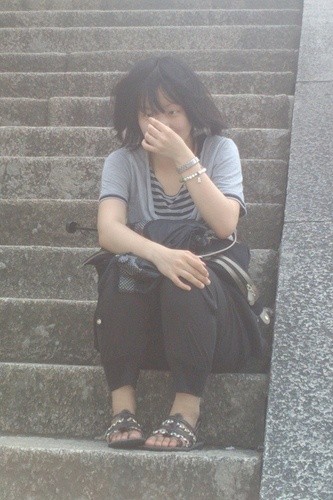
[138,219,275,335]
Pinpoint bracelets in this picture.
[176,156,207,183]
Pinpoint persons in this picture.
[94,57,264,449]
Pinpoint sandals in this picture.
[146,415,205,449]
[104,408,146,448]
